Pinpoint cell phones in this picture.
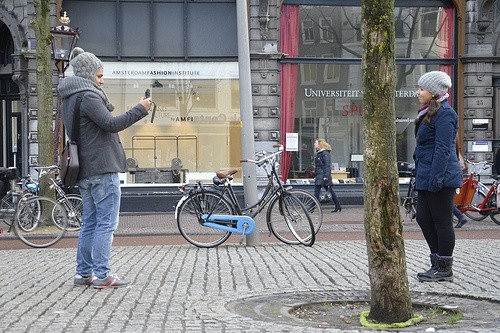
[145,89,150,99]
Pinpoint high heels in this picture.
[306,208,314,213]
[331,206,342,212]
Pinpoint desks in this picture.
[128,168,189,183]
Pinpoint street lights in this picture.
[50,11,81,199]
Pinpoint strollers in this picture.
[400,170,418,220]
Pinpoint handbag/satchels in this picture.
[319,180,332,203]
[60,141,79,186]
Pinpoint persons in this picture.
[307,139,341,213]
[452,143,468,228]
[57,47,151,288]
[412,71,463,280]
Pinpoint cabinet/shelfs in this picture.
[331,172,350,179]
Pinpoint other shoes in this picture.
[455,217,467,228]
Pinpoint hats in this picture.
[417,71,452,99]
[70,47,114,111]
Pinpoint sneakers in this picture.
[73,273,97,289]
[93,272,127,288]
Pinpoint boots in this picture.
[419,255,453,281]
[418,253,439,278]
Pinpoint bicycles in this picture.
[0,165,84,249]
[453,158,500,226]
[173,144,324,249]
